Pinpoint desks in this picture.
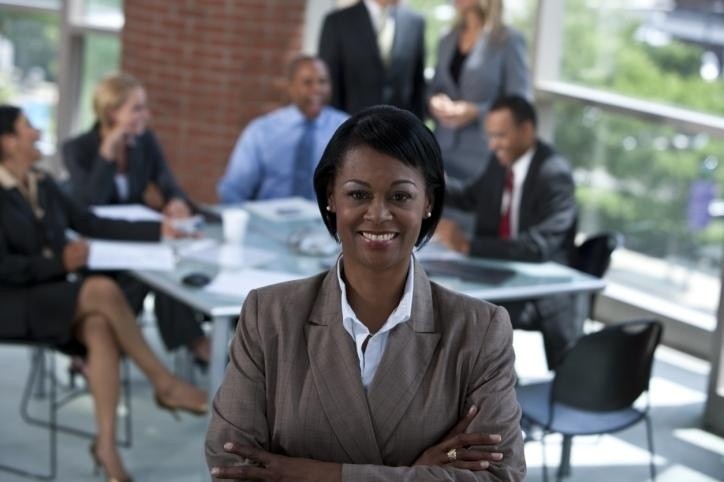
[128,197,604,422]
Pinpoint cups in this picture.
[221,207,250,247]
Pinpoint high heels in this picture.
[90,441,132,482]
[153,393,210,420]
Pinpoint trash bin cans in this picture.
[690,183,711,227]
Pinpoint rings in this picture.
[446,446,458,462]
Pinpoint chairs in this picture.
[0,345,133,481]
[512,231,617,331]
[514,318,664,482]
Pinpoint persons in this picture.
[0,103,210,482]
[60,69,210,391]
[435,93,589,371]
[203,105,528,481]
[315,0,427,123]
[214,51,354,334]
[424,0,531,229]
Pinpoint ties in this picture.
[498,170,514,239]
[292,120,317,199]
